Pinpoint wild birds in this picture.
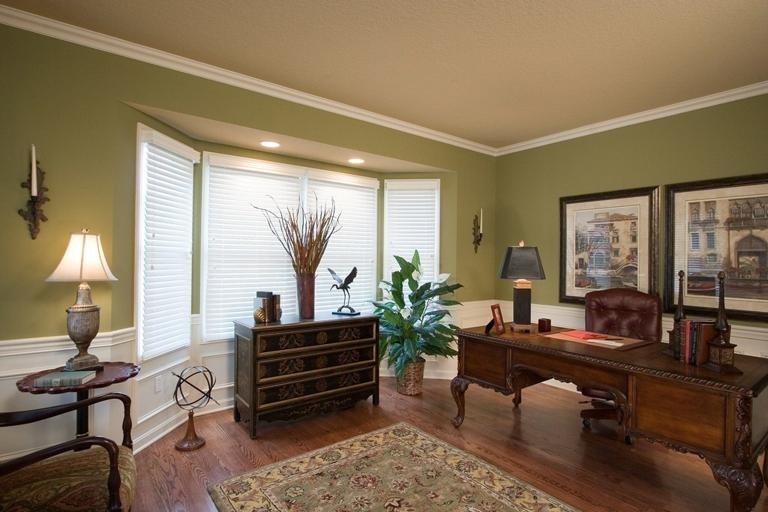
[327,267,357,307]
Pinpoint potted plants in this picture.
[372,248,466,396]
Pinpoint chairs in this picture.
[1,392,138,510]
[579,286,662,444]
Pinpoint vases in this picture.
[295,271,315,320]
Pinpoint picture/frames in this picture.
[663,173,767,323]
[485,304,506,335]
[559,184,661,305]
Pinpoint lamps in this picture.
[500,241,546,332]
[42,227,120,371]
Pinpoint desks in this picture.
[449,322,768,511]
[16,361,141,454]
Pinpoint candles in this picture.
[479,207,484,234]
[30,142,39,195]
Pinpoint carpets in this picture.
[206,421,585,511]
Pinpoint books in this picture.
[561,330,607,340]
[254,291,280,324]
[33,370,97,387]
[679,318,718,367]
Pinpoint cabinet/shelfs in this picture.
[232,308,380,439]
